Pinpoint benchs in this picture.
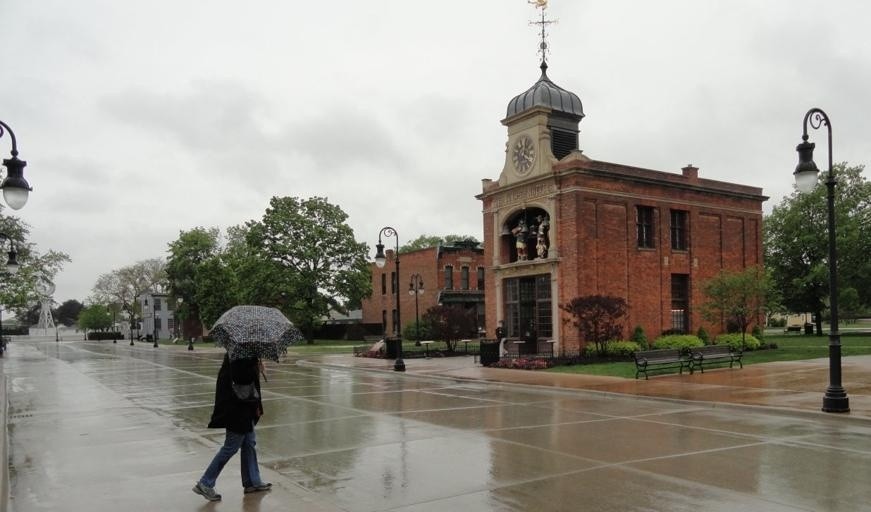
[632,349,694,380]
[683,344,743,373]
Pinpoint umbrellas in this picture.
[207,305,304,381]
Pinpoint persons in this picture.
[516,218,529,261]
[535,215,546,258]
[192,350,272,502]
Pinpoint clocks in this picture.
[508,132,535,178]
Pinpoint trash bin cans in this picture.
[386,336,402,357]
[480,339,500,366]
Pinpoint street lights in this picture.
[408,273,425,347]
[1,231,21,277]
[375,226,407,371]
[792,107,851,413]
[0,120,34,217]
[107,296,197,351]
